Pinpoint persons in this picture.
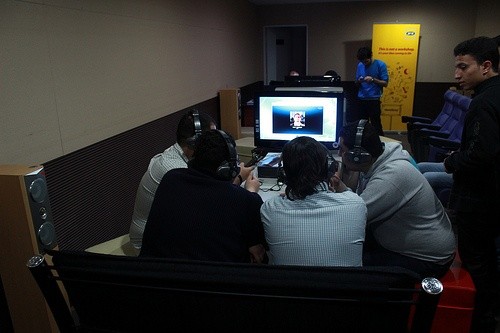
[443,36,500,333]
[128,109,257,258]
[354,46,389,137]
[414,160,461,192]
[324,71,338,78]
[259,136,368,268]
[137,129,269,265]
[287,68,302,77]
[328,119,459,333]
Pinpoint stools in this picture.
[407,267,476,333]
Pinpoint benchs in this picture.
[27,249,443,333]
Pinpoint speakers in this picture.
[220,88,242,140]
[0,163,72,333]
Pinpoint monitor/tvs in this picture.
[284,75,341,82]
[254,90,344,151]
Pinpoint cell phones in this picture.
[244,154,261,167]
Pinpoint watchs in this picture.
[370,76,374,82]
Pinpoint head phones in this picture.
[186,108,202,150]
[188,129,235,181]
[277,136,339,186]
[347,119,371,164]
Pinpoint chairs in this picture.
[402,90,472,162]
